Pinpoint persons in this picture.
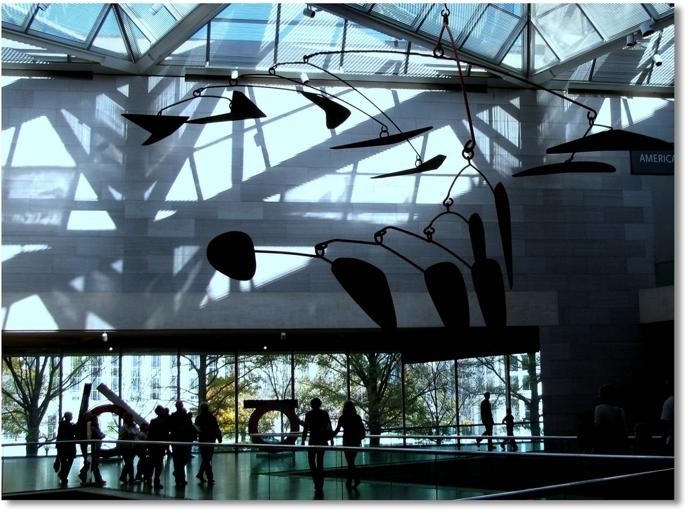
[333,400,366,489]
[631,421,654,456]
[592,383,627,453]
[476,391,496,451]
[53,400,223,489]
[502,407,519,451]
[660,394,674,456]
[303,397,335,492]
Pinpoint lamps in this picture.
[299,73,309,85]
[302,6,316,18]
[651,54,663,67]
[625,33,635,47]
